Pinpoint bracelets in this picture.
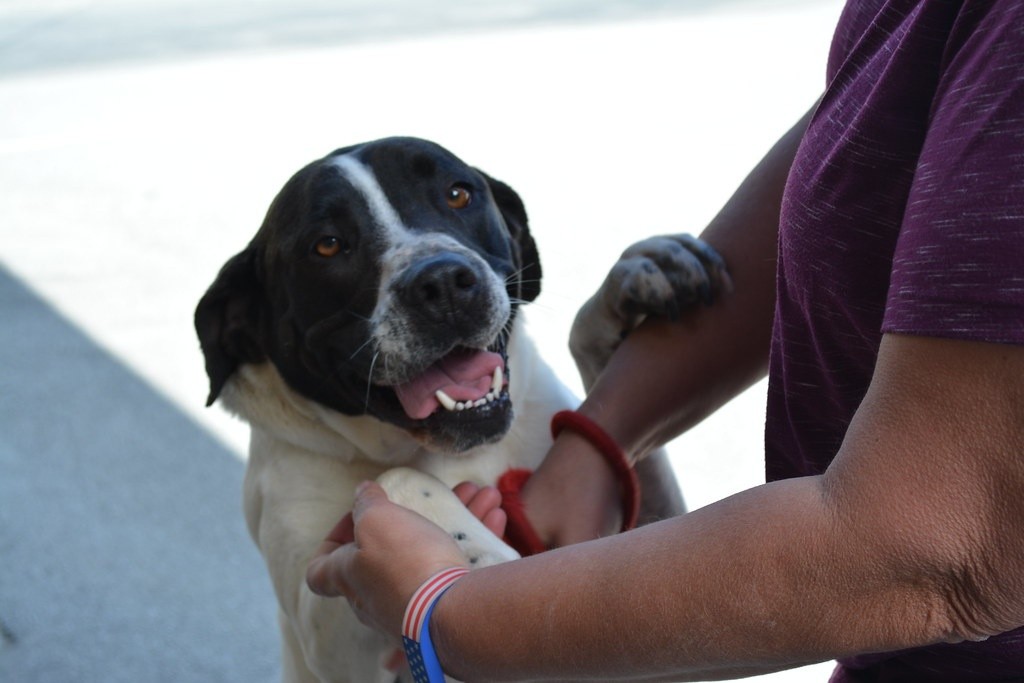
[400,567,470,682]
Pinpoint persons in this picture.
[303,1,1024,683]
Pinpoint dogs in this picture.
[193,136,728,683]
